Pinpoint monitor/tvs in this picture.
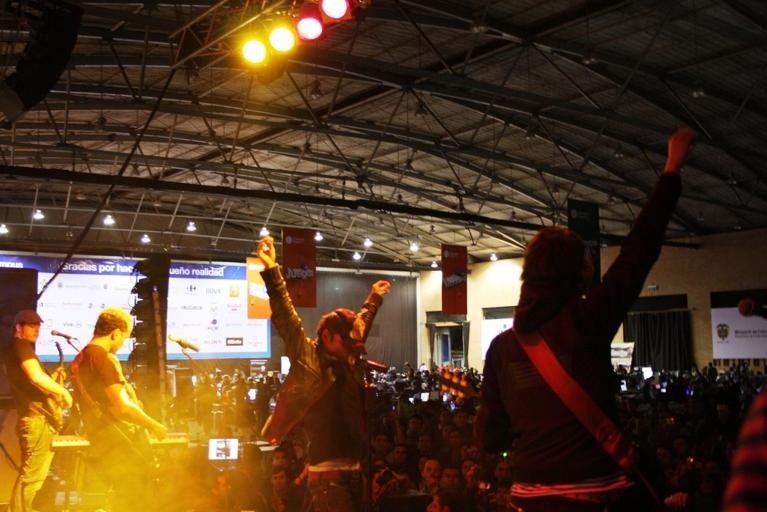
[207,438,240,463]
[187,363,694,420]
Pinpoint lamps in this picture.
[294,1,369,43]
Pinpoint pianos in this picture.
[49,432,190,453]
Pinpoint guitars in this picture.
[43,341,64,432]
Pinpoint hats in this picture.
[330,307,367,356]
[16,310,43,325]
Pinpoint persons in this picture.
[472,124,694,511]
[8,309,73,512]
[67,308,168,512]
[190,361,767,512]
[254,237,390,512]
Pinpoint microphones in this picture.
[50,329,80,343]
[168,333,202,355]
